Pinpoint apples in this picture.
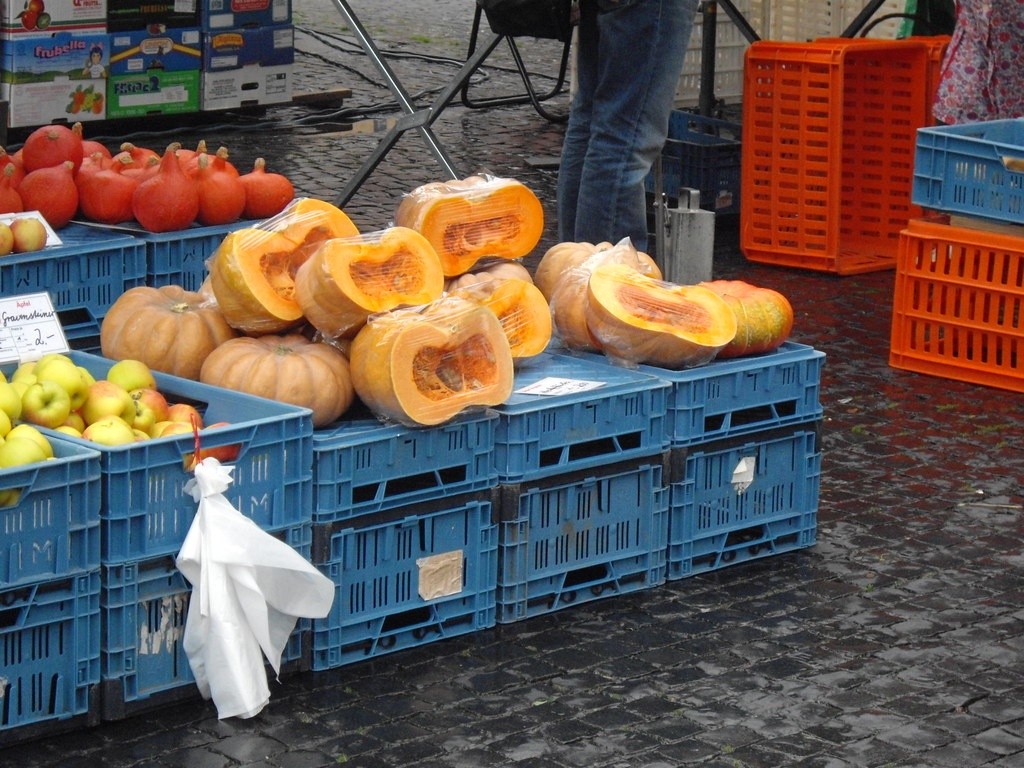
[0,217,47,256]
[0,353,240,508]
[22,0,45,30]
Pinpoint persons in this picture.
[933,0,1024,125]
[559,0,698,253]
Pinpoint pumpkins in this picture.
[0,122,296,233]
[97,175,793,429]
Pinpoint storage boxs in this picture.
[499,464,671,623]
[112,217,276,294]
[1,223,147,341]
[740,37,928,276]
[313,408,500,523]
[314,499,499,671]
[668,430,822,580]
[911,119,1024,224]
[568,340,827,447]
[0,0,294,128]
[644,110,741,217]
[907,35,953,128]
[888,218,1024,392]
[0,422,105,749]
[0,348,315,722]
[496,351,672,482]
